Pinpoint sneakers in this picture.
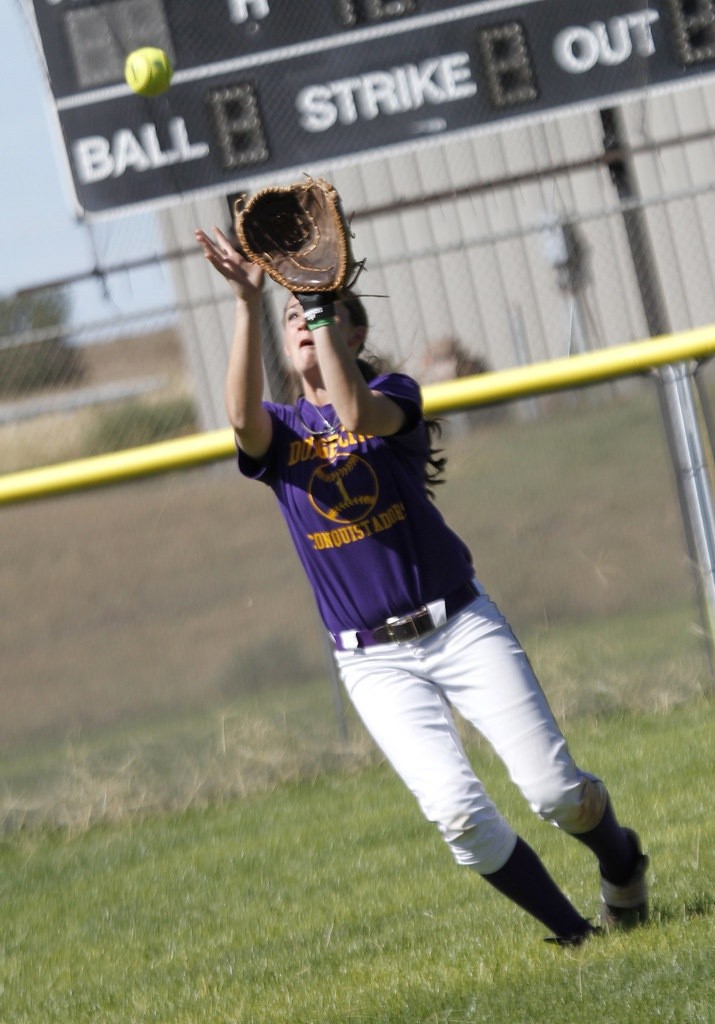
[543,924,595,953]
[598,829,649,931]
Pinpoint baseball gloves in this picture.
[231,176,365,295]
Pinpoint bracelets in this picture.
[305,315,336,331]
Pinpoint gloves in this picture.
[293,289,335,325]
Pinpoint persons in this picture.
[196,176,651,950]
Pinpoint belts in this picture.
[334,579,480,653]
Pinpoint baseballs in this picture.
[122,45,172,100]
